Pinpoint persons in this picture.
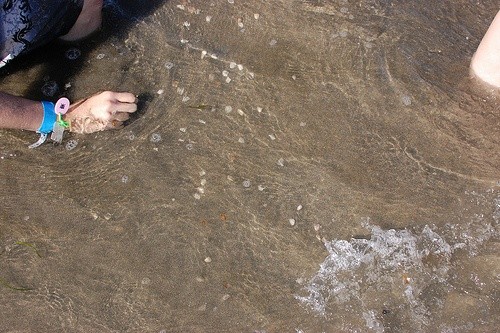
[0,0,139,147]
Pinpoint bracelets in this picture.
[26,98,56,148]
